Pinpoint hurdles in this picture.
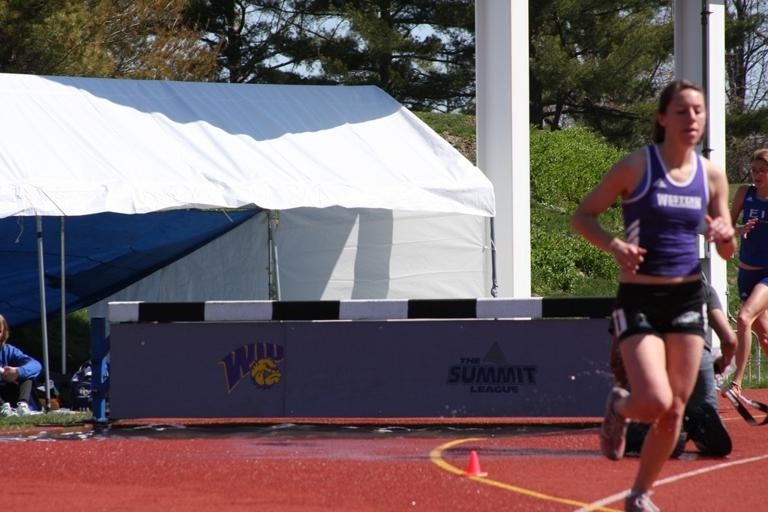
[106,297,616,434]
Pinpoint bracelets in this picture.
[721,237,733,243]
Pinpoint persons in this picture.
[721,146,768,402]
[608,278,739,458]
[68,359,95,411]
[570,76,738,511]
[0,315,44,418]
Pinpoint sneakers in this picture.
[0,401,35,418]
[721,382,741,399]
[600,384,632,461]
[624,491,661,512]
